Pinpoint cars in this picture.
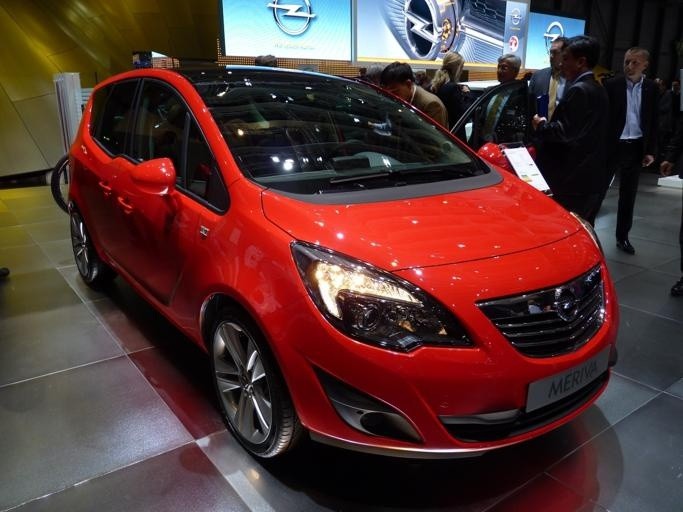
[66,62,624,477]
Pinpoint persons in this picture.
[659,112,682,296]
[359,36,680,254]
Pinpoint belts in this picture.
[619,139,642,144]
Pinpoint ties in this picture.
[548,73,561,122]
[482,90,506,140]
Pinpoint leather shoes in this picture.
[671,276,683,296]
[615,236,635,254]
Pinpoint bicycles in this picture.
[48,152,71,216]
